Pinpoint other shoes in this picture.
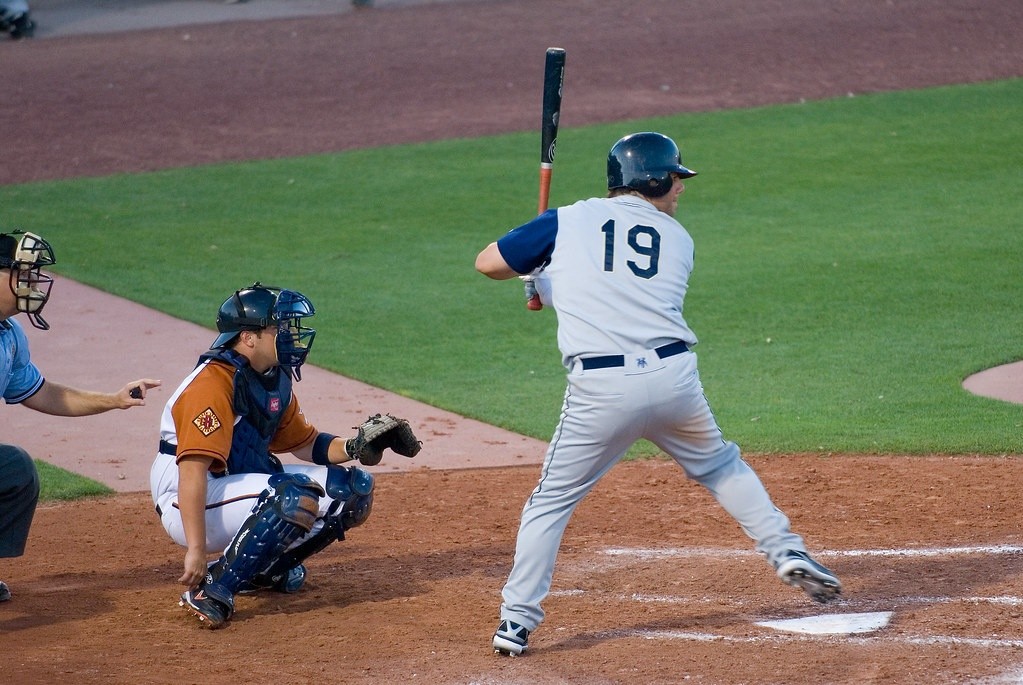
[9,12,36,39]
[0,581,11,600]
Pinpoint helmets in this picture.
[606,131,699,198]
[209,285,278,350]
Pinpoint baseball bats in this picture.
[526,47,567,311]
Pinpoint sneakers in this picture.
[772,548,842,604]
[492,620,530,657]
[239,564,307,593]
[178,583,229,629]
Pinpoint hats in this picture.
[0,233,57,269]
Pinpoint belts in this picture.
[581,340,689,371]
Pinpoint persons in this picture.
[0,232,161,601]
[150,283,421,627]
[474,132,841,657]
[0,0,37,38]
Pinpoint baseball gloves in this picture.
[344,413,423,466]
